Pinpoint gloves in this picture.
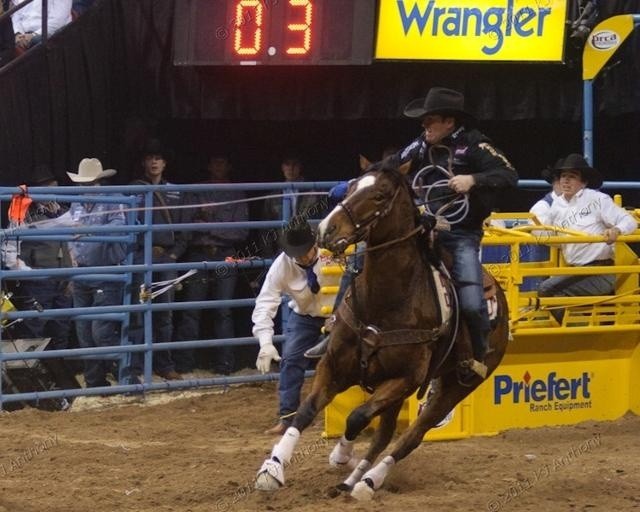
[256,343,282,376]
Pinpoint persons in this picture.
[127,147,193,385]
[0,164,74,350]
[529,157,563,213]
[9,0,72,47]
[31,157,139,398]
[305,86,519,385]
[267,159,318,219]
[251,222,341,433]
[528,151,637,323]
[172,156,251,376]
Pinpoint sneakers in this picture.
[263,421,292,435]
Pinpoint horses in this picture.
[253,154,508,502]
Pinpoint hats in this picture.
[275,216,318,259]
[66,158,117,183]
[20,162,59,185]
[403,88,465,119]
[540,153,603,190]
